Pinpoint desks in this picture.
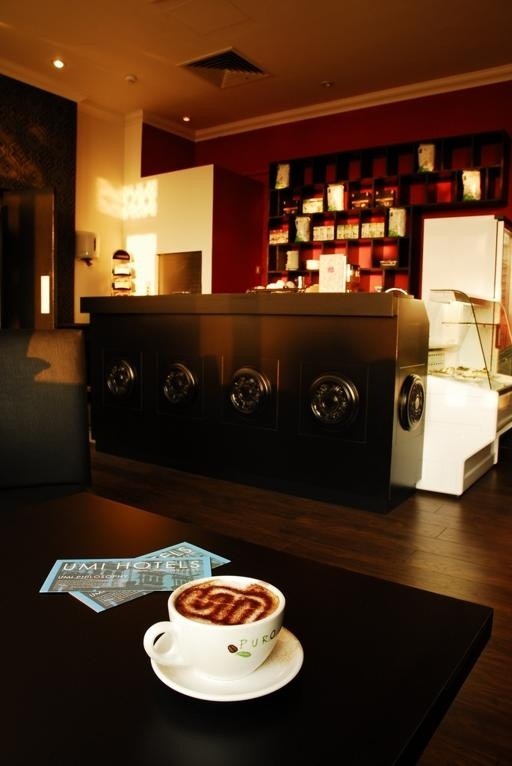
[0,492,495,765]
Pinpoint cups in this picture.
[143,575,286,678]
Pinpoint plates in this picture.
[149,626,304,702]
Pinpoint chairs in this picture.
[0,328,91,514]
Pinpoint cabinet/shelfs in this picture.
[266,130,512,299]
